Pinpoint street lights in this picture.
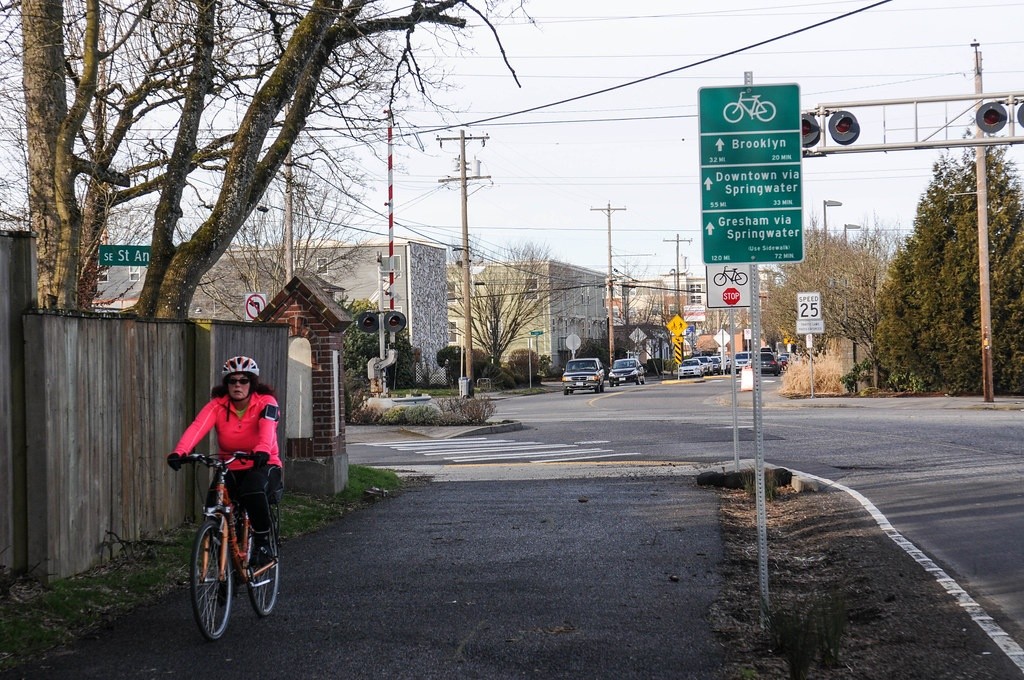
[824,200,842,240]
[844,224,860,245]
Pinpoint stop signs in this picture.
[723,289,740,305]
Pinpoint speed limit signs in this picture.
[797,292,822,321]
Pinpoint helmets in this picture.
[222,356,259,380]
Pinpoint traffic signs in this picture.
[698,85,804,265]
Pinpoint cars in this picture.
[691,356,714,376]
[679,359,705,379]
[608,358,645,387]
[779,356,787,361]
[756,353,778,376]
[710,357,723,375]
[718,356,731,374]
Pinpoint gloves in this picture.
[167,453,181,471]
[253,452,270,469]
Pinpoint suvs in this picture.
[760,347,774,355]
[734,351,753,374]
[562,358,604,395]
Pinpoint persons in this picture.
[168,356,283,607]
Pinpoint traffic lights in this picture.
[384,311,406,333]
[802,111,860,148]
[358,312,379,332]
[976,99,1024,133]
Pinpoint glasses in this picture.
[228,379,250,384]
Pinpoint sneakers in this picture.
[218,575,238,606]
[250,546,272,566]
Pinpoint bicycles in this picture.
[166,449,280,641]
